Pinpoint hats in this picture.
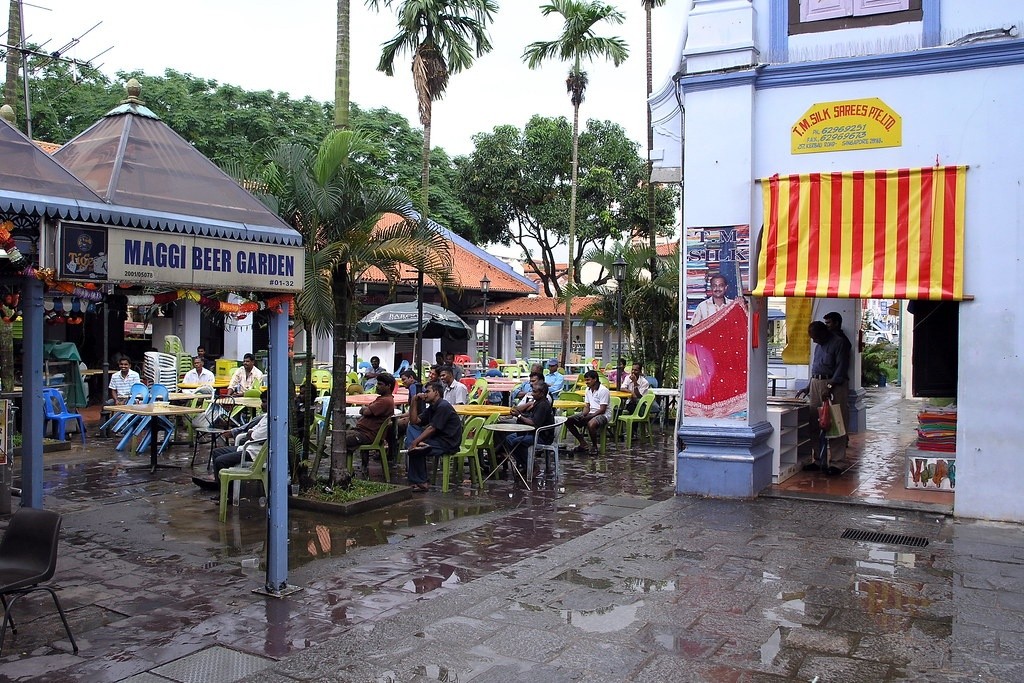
[549,359,558,366]
[490,359,498,369]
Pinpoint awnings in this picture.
[886,302,899,316]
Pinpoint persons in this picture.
[609,358,628,383]
[439,366,470,421]
[545,358,564,400]
[435,351,462,382]
[406,382,462,491]
[513,364,552,412]
[795,312,852,476]
[485,361,503,402]
[428,365,447,390]
[347,356,426,460]
[620,362,649,438]
[505,382,554,477]
[573,335,582,347]
[565,370,612,457]
[101,346,267,504]
[692,275,748,328]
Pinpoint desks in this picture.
[649,388,679,430]
[347,392,410,406]
[767,376,795,396]
[577,382,618,390]
[574,390,632,439]
[205,397,262,419]
[551,400,586,455]
[482,376,521,384]
[117,392,211,450]
[562,375,578,381]
[457,363,481,368]
[498,364,518,372]
[487,383,521,407]
[102,402,206,473]
[564,363,593,374]
[450,405,512,473]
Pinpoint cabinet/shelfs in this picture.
[766,404,816,484]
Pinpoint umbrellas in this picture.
[356,300,473,370]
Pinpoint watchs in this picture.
[827,384,832,389]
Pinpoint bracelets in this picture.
[518,414,521,418]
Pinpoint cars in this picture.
[862,329,894,347]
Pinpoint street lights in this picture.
[480,272,491,373]
[610,255,629,391]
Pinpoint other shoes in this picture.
[645,431,650,438]
[520,467,540,479]
[631,435,639,441]
[824,466,842,476]
[210,493,233,505]
[802,463,828,471]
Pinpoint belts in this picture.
[812,375,833,380]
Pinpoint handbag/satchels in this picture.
[817,399,832,432]
[824,399,846,439]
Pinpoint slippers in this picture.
[588,446,598,456]
[572,446,589,453]
[410,483,429,492]
[408,443,432,456]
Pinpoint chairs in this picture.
[99,333,659,521]
[0,508,79,653]
[42,388,88,444]
